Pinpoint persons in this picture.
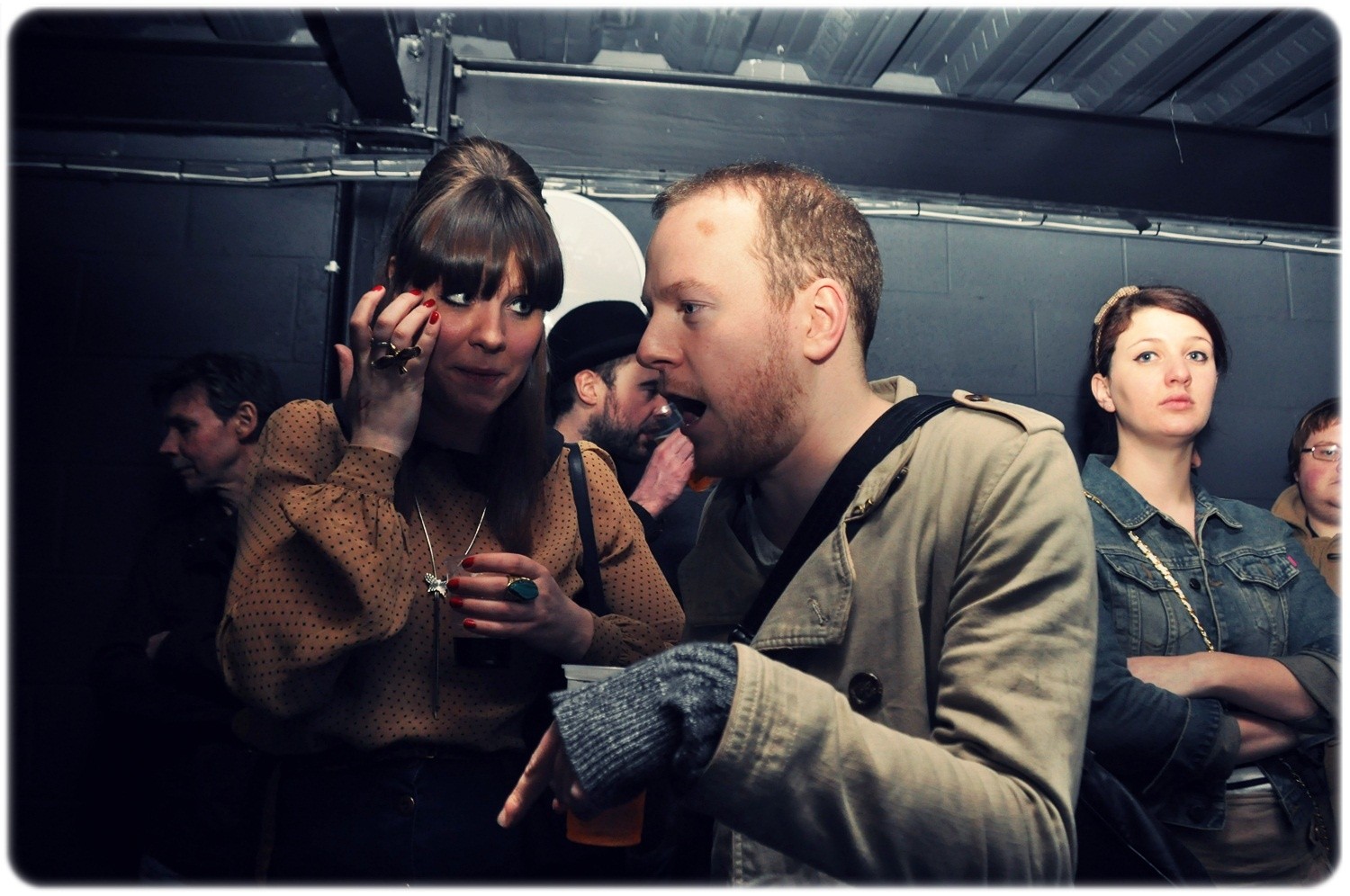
[617,408,718,589]
[91,350,288,880]
[495,163,1100,884]
[537,300,695,545]
[1271,398,1343,598]
[216,136,687,882]
[1080,288,1342,885]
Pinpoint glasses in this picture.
[1302,441,1339,462]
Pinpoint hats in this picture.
[546,301,650,383]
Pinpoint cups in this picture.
[640,401,713,492]
[562,663,643,847]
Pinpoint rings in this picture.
[370,341,421,375]
[369,336,389,348]
[503,575,539,603]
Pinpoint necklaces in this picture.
[403,456,489,718]
